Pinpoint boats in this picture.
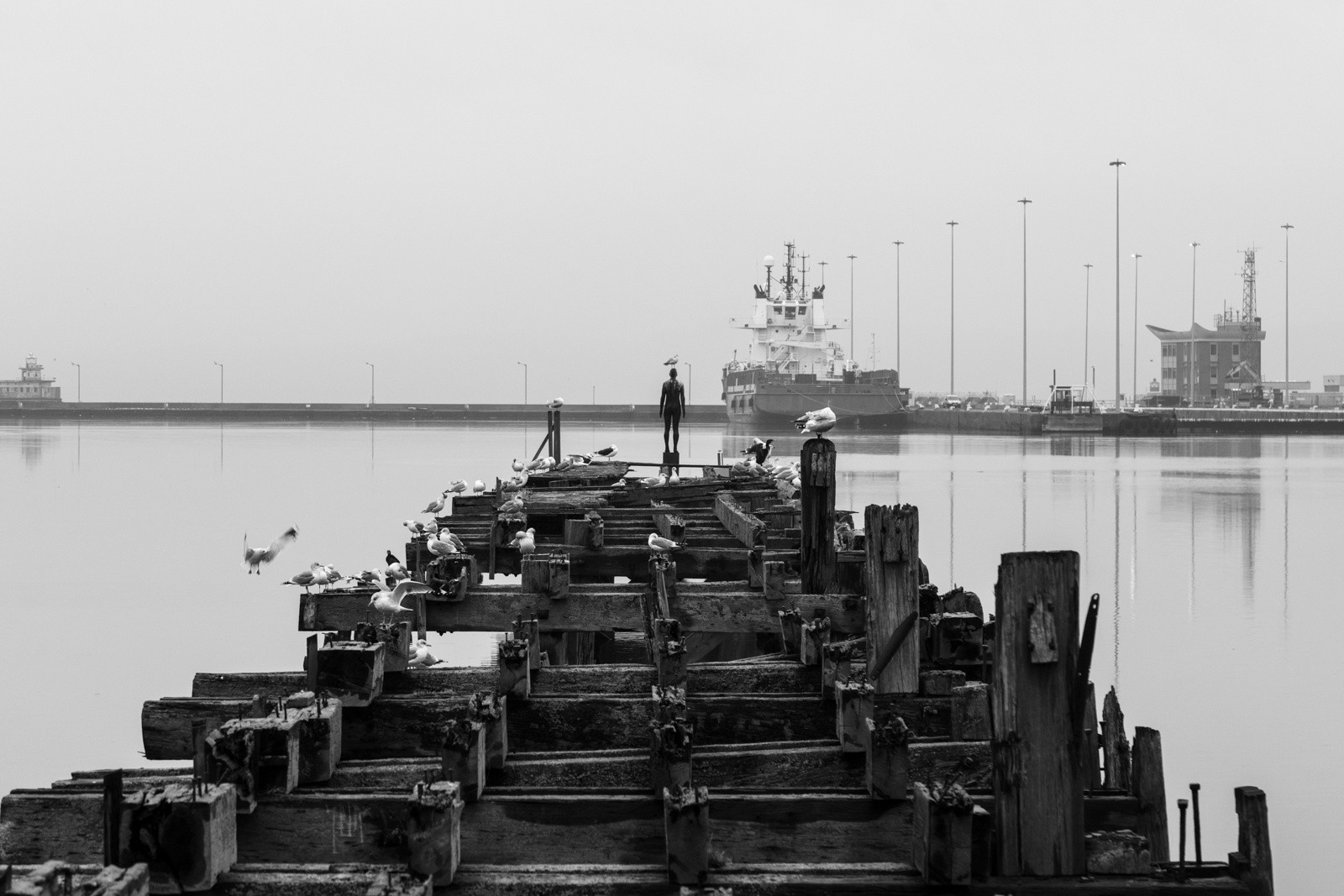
[720,238,913,426]
[0,351,63,403]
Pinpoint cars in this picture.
[943,395,962,409]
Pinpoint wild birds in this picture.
[497,495,524,513]
[368,577,436,628]
[508,527,536,556]
[500,444,618,493]
[740,439,802,490]
[444,480,468,497]
[612,479,626,485]
[426,528,468,558]
[242,524,302,577]
[791,406,838,439]
[403,518,439,538]
[281,559,341,594]
[474,481,487,497]
[420,492,449,518]
[648,532,689,555]
[636,467,681,488]
[345,549,412,586]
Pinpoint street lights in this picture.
[517,362,527,403]
[762,254,775,298]
[365,362,375,404]
[847,253,858,361]
[1129,252,1142,407]
[1108,158,1126,412]
[892,239,905,387]
[1016,197,1033,408]
[946,219,959,394]
[214,362,224,403]
[1190,241,1201,407]
[1081,262,1094,384]
[70,361,81,402]
[1280,221,1295,406]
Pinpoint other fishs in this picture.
[410,637,447,669]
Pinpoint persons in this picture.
[659,368,685,452]
